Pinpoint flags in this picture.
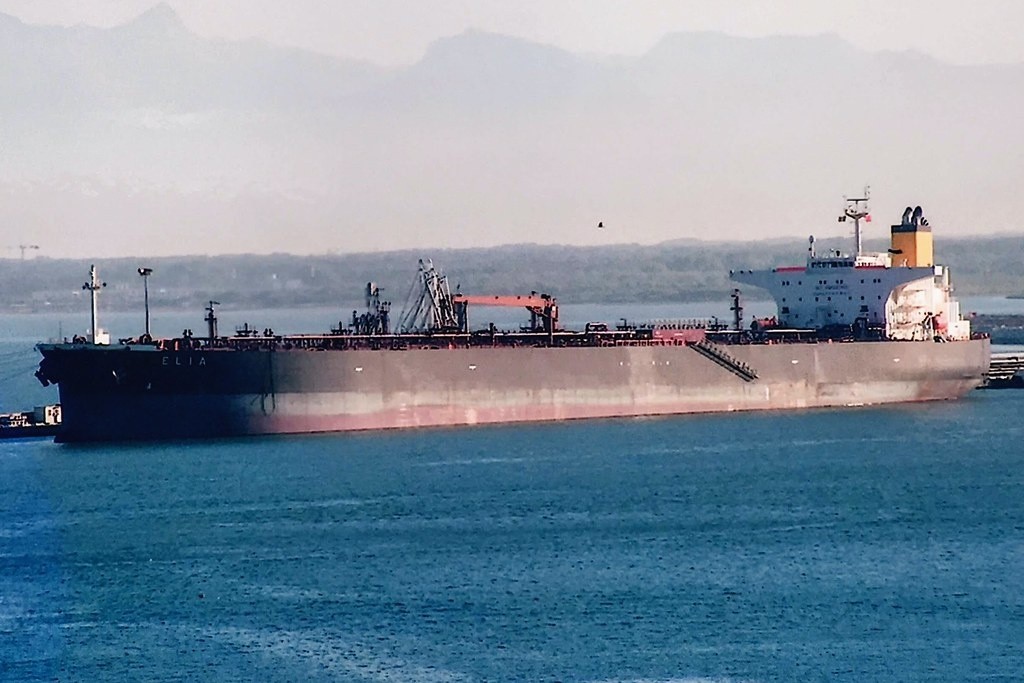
[838,216,846,222]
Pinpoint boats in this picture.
[34,182,991,445]
[0,404,63,438]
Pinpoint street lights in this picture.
[138,266,155,335]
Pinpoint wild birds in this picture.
[598,221,605,228]
